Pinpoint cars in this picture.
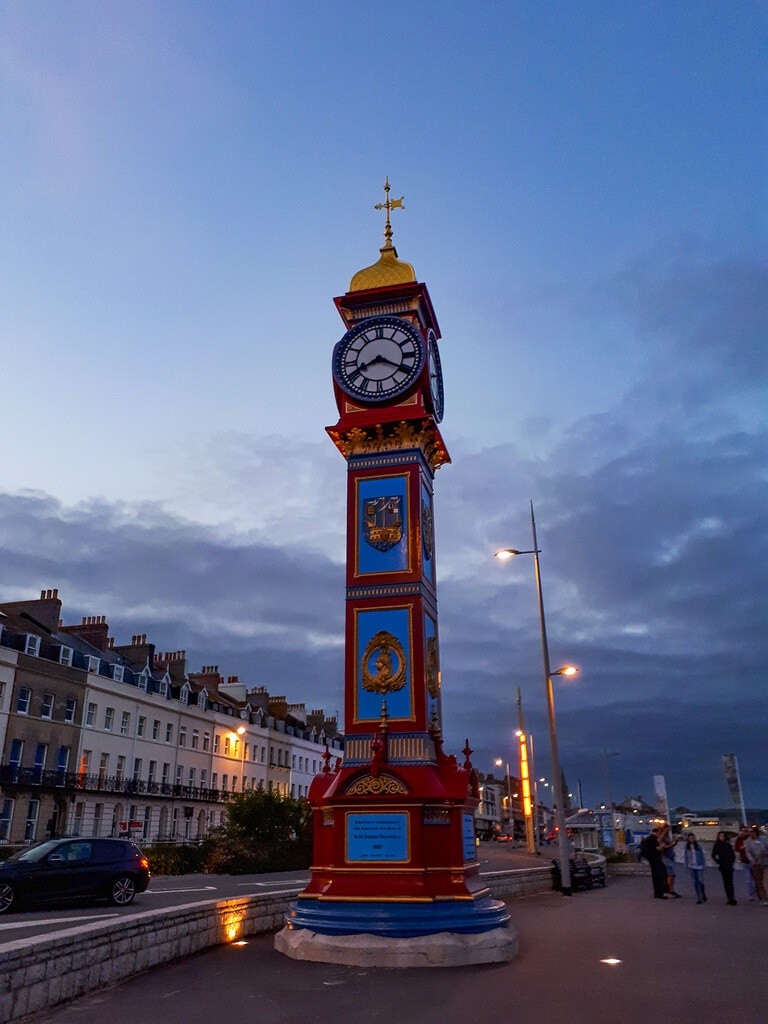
[0,837,151,914]
[497,834,508,842]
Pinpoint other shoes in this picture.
[749,897,754,901]
[696,896,708,904]
[654,895,668,900]
[760,902,768,908]
[727,900,737,905]
[668,891,682,898]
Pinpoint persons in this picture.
[684,832,708,904]
[712,830,737,905]
[635,823,681,899]
[734,826,768,901]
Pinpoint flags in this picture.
[653,775,669,815]
[723,754,742,803]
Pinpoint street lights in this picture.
[237,727,246,793]
[516,731,545,855]
[494,548,579,898]
[495,760,519,849]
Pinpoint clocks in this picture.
[332,316,425,401]
[427,330,445,423]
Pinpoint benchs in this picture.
[551,857,607,893]
